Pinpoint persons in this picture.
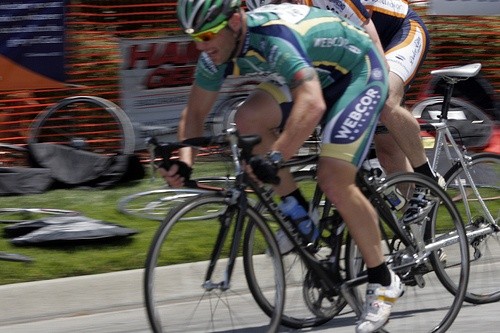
[158,0,408,333]
[245,0,448,225]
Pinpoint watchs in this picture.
[266,151,284,163]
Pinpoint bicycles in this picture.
[117,174,248,221]
[142,125,470,333]
[242,62,500,327]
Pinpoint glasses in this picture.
[189,21,228,42]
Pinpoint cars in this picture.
[408,97,499,148]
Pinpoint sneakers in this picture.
[356,270,404,333]
[266,202,319,258]
[403,170,447,224]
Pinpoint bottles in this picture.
[279,196,319,241]
[380,179,405,210]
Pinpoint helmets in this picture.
[246,0,271,10]
[177,0,242,34]
